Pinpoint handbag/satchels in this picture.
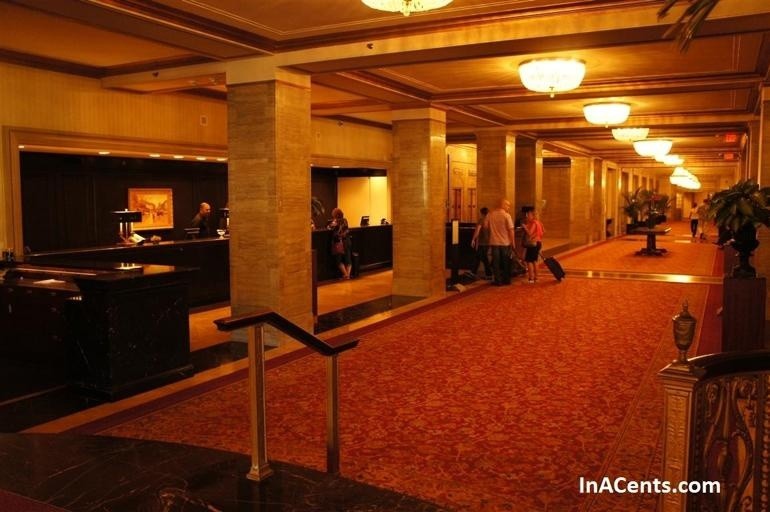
[331,240,345,256]
[522,238,538,248]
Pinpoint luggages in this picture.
[543,256,565,283]
[508,251,527,275]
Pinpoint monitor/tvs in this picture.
[360,215,369,226]
[183,228,200,240]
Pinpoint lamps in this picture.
[359,0,457,19]
[509,55,704,197]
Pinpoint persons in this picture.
[326,207,353,280]
[484,198,517,286]
[219,201,230,233]
[190,201,211,239]
[688,202,701,237]
[520,208,543,284]
[471,206,492,279]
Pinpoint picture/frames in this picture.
[126,183,178,233]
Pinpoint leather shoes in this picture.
[490,279,511,286]
[525,278,539,283]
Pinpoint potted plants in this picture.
[683,178,770,280]
[616,184,670,228]
